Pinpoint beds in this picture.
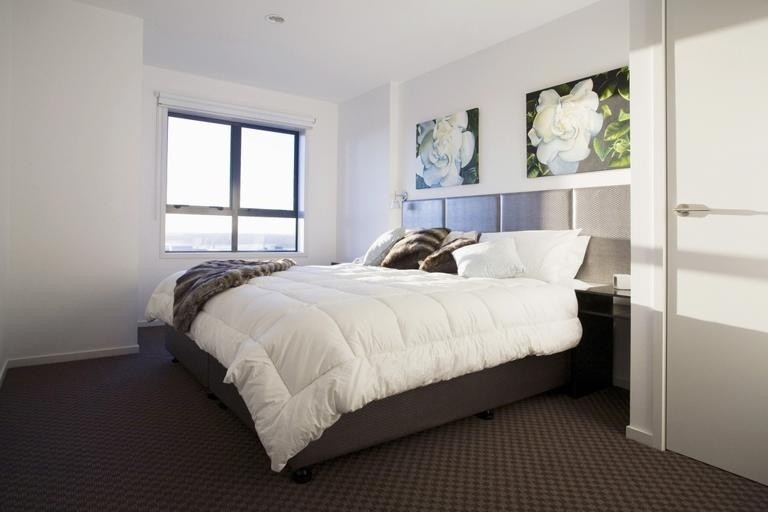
[143,184,630,485]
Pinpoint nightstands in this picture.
[575,285,630,391]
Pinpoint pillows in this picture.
[363,226,526,282]
